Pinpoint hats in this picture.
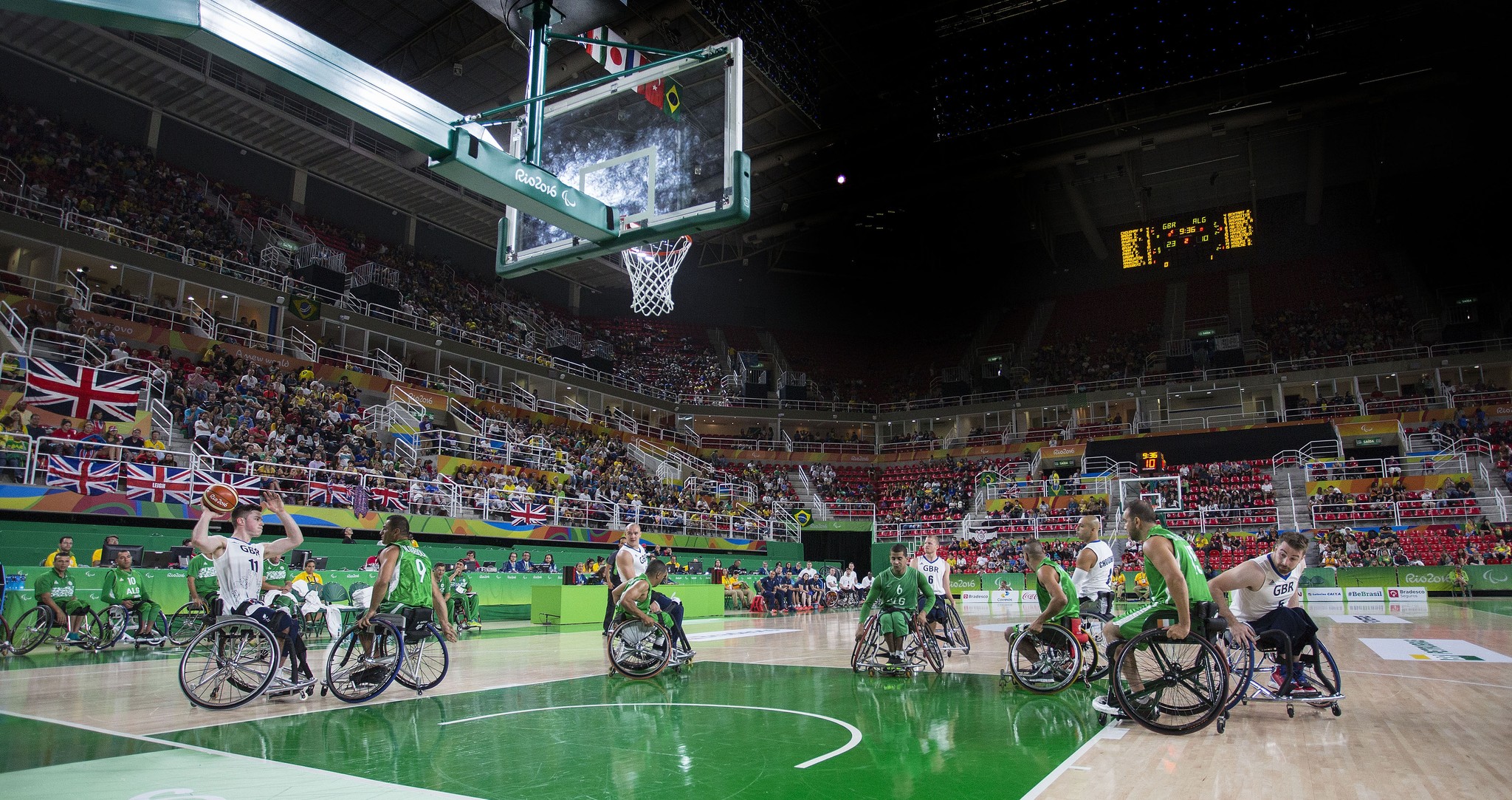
[456,559,466,567]
[109,426,118,431]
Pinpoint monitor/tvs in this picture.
[290,549,312,570]
[532,563,551,573]
[483,561,496,567]
[587,577,599,585]
[688,562,702,574]
[99,545,195,569]
[462,560,476,572]
[667,564,676,573]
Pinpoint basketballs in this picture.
[202,483,240,513]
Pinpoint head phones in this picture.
[508,552,518,560]
[1456,570,1462,575]
[650,556,657,560]
[182,538,192,545]
[587,557,592,563]
[467,550,475,558]
[549,554,554,564]
[670,554,677,562]
[598,557,603,563]
[522,551,531,560]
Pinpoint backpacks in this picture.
[750,595,764,613]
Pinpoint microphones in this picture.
[1341,568,1349,570]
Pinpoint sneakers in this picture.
[1293,662,1317,692]
[146,632,155,639]
[894,656,906,670]
[771,604,824,613]
[928,638,934,646]
[885,654,895,671]
[467,621,476,626]
[133,633,149,639]
[742,605,750,610]
[68,633,81,641]
[1127,697,1153,711]
[1023,665,1055,684]
[1267,665,1304,693]
[733,606,739,610]
[472,618,482,626]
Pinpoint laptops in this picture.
[313,556,328,570]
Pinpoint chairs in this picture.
[0,117,1512,638]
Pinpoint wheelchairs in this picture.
[1043,591,1119,686]
[1090,600,1232,736]
[321,607,450,705]
[606,610,697,680]
[7,600,105,656]
[999,614,1100,695]
[0,613,11,656]
[432,598,481,633]
[178,598,318,713]
[823,588,882,608]
[1191,618,1346,733]
[850,609,944,676]
[901,603,971,656]
[258,594,307,639]
[168,593,217,647]
[84,602,169,650]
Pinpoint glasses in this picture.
[109,540,119,543]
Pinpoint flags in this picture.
[126,462,192,505]
[510,500,547,527]
[368,486,409,510]
[309,480,355,505]
[45,455,120,497]
[979,472,997,487]
[22,355,144,422]
[791,508,814,527]
[1048,485,1065,496]
[581,26,685,122]
[193,470,261,509]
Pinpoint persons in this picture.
[854,533,958,673]
[611,559,675,656]
[187,497,459,689]
[1002,499,1328,712]
[0,124,1512,656]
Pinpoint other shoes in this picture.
[269,669,293,687]
[602,630,608,636]
